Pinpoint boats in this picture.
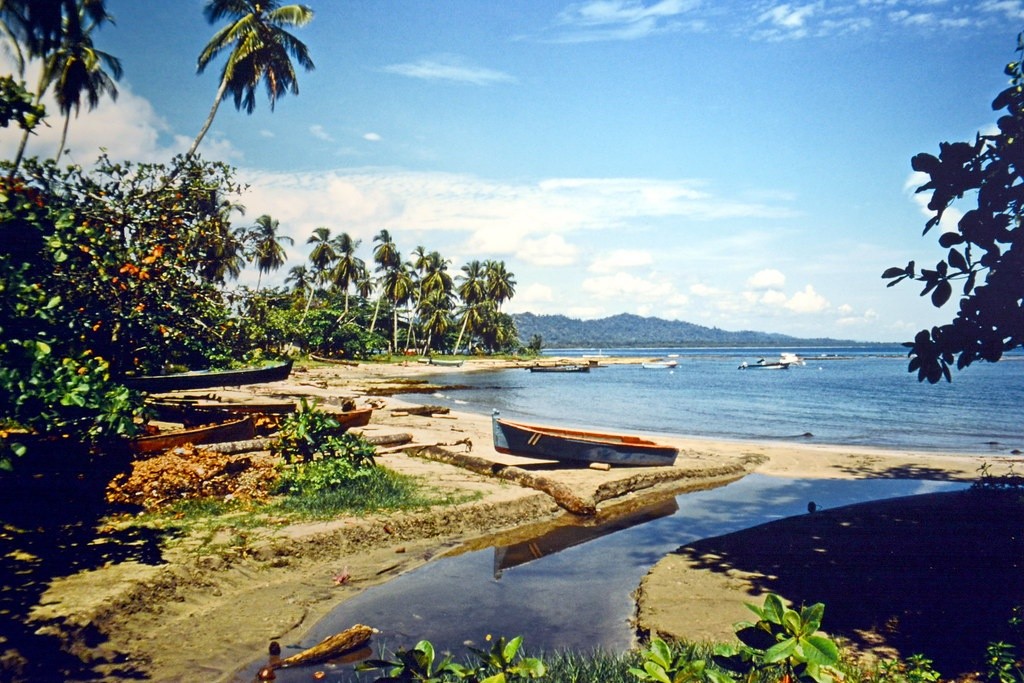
[117,358,295,392]
[737,359,791,369]
[417,357,464,366]
[526,362,590,373]
[0,397,374,458]
[642,360,677,370]
[490,408,680,467]
[310,354,360,368]
